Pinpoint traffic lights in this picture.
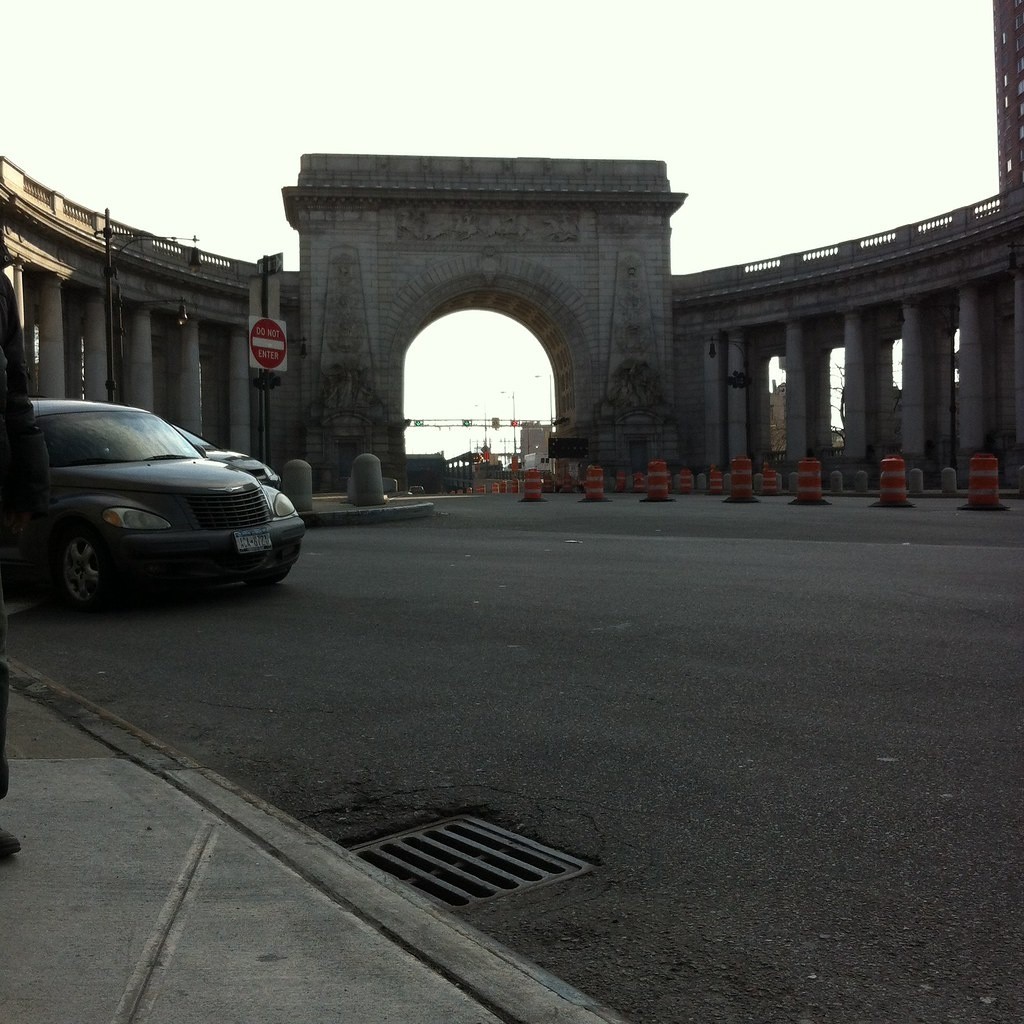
[415,420,422,426]
[463,420,470,427]
[511,421,518,427]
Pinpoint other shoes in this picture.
[0,826,21,855]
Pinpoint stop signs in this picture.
[249,314,288,371]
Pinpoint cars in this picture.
[32,398,306,614]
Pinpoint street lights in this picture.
[535,375,552,470]
[706,336,755,471]
[500,390,517,452]
[475,404,487,445]
[93,208,203,405]
[910,302,958,469]
[114,284,189,404]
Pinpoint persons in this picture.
[0,273,50,853]
